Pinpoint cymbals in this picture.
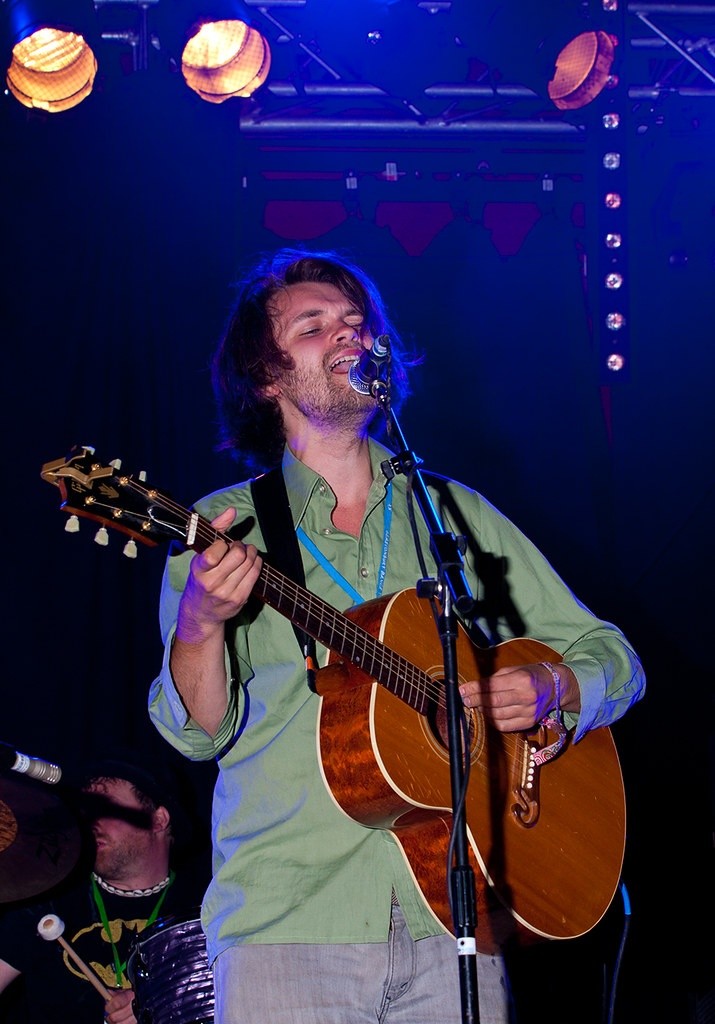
[0,767,81,902]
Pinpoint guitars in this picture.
[39,442,626,959]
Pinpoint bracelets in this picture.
[532,662,566,765]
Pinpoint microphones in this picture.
[1,744,62,788]
[348,335,390,397]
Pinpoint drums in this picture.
[125,902,217,1024]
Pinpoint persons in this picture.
[148,251,649,1024]
[1,761,184,1024]
